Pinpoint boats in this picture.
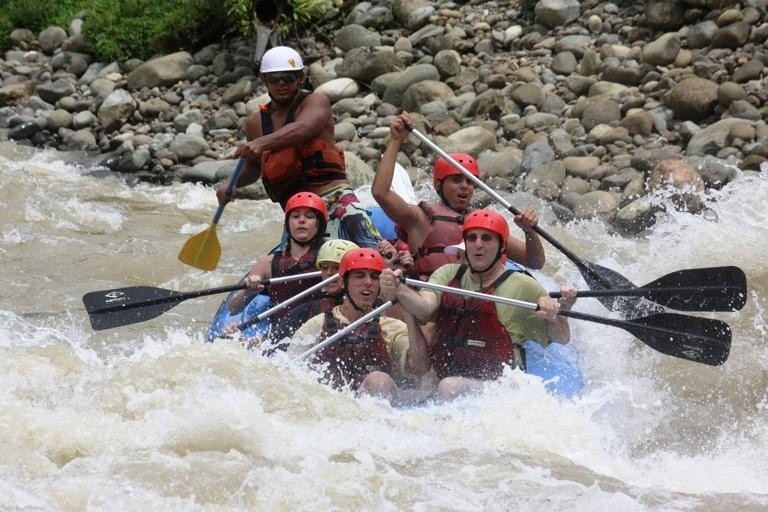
[210,206,582,405]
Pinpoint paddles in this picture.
[399,277,731,367]
[549,265,747,311]
[402,118,667,320]
[177,157,249,271]
[83,270,322,331]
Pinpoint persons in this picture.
[226,152,578,407]
[215,45,384,252]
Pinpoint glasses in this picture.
[466,233,493,241]
[266,73,296,83]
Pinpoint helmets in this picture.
[434,153,479,181]
[260,46,304,73]
[316,239,384,277]
[463,210,509,252]
[285,192,329,233]
